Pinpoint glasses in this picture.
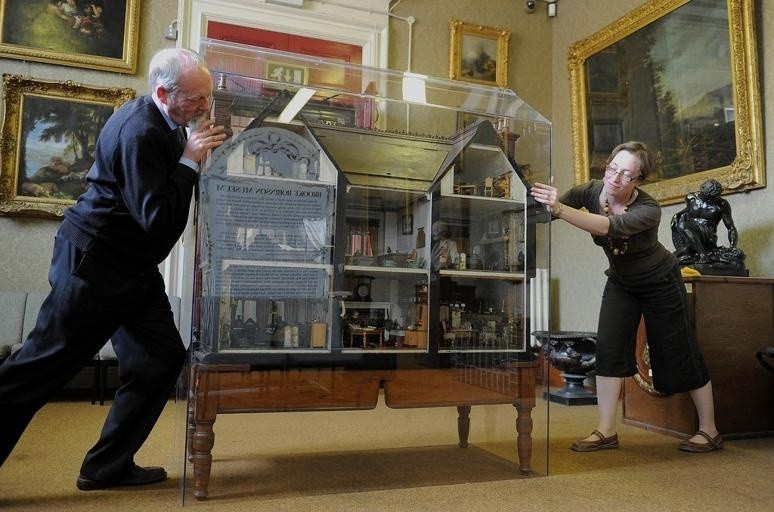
[604,164,643,182]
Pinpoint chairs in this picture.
[0,290,28,354]
[100,294,181,367]
[10,292,98,405]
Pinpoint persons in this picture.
[431,220,459,271]
[668,179,737,265]
[531,141,724,454]
[0,46,229,491]
[47,0,104,38]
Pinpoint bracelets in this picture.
[555,203,564,218]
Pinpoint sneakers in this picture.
[678,430,722,453]
[76,466,168,490]
[571,430,618,452]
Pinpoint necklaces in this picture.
[603,194,630,256]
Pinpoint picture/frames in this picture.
[456,103,510,150]
[566,0,766,207]
[447,17,511,87]
[0,72,136,217]
[0,0,141,74]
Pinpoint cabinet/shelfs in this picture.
[182,88,545,499]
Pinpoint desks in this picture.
[606,275,774,443]
